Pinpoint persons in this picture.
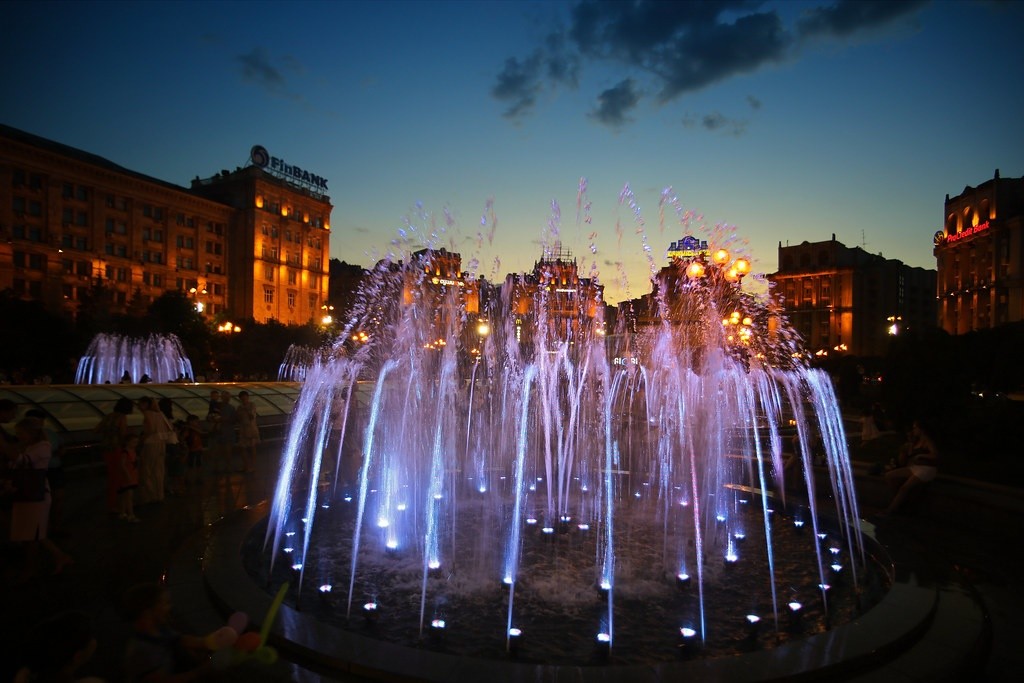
[774,407,940,522]
[0,388,259,683]
[291,374,635,488]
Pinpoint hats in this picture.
[24,409,46,419]
[159,398,174,405]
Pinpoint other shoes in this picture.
[237,464,246,473]
[127,515,141,522]
[118,513,131,519]
[245,465,255,473]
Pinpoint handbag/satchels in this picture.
[160,412,179,444]
[3,454,44,501]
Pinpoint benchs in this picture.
[180,415,284,461]
[61,425,145,458]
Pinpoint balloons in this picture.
[205,582,289,672]
